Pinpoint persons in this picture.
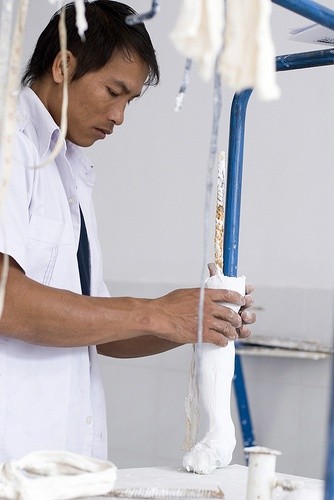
[0,0,257,465]
